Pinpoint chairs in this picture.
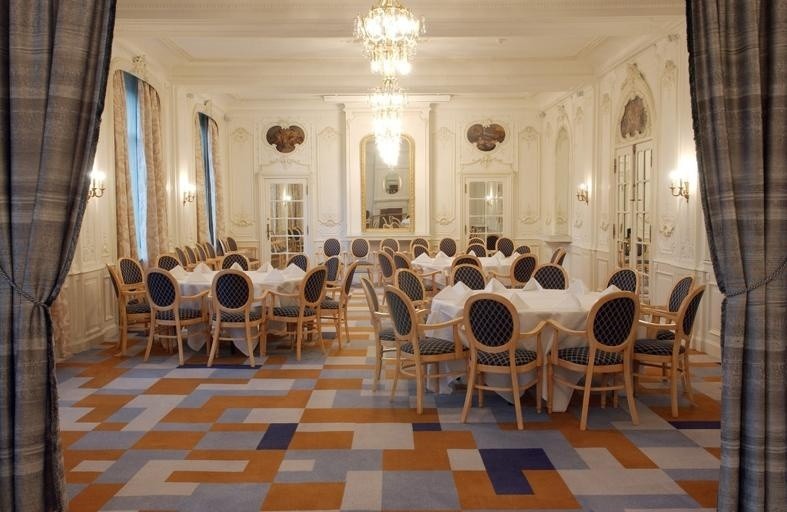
[544,291,642,432]
[641,274,696,380]
[360,274,429,392]
[103,238,567,368]
[607,268,639,294]
[631,284,707,419]
[387,284,474,414]
[460,293,548,431]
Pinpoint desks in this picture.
[427,285,620,412]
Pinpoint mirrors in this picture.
[361,131,414,231]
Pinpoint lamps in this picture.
[368,77,414,115]
[576,181,590,207]
[664,171,691,203]
[183,182,198,207]
[351,1,425,76]
[84,170,108,201]
